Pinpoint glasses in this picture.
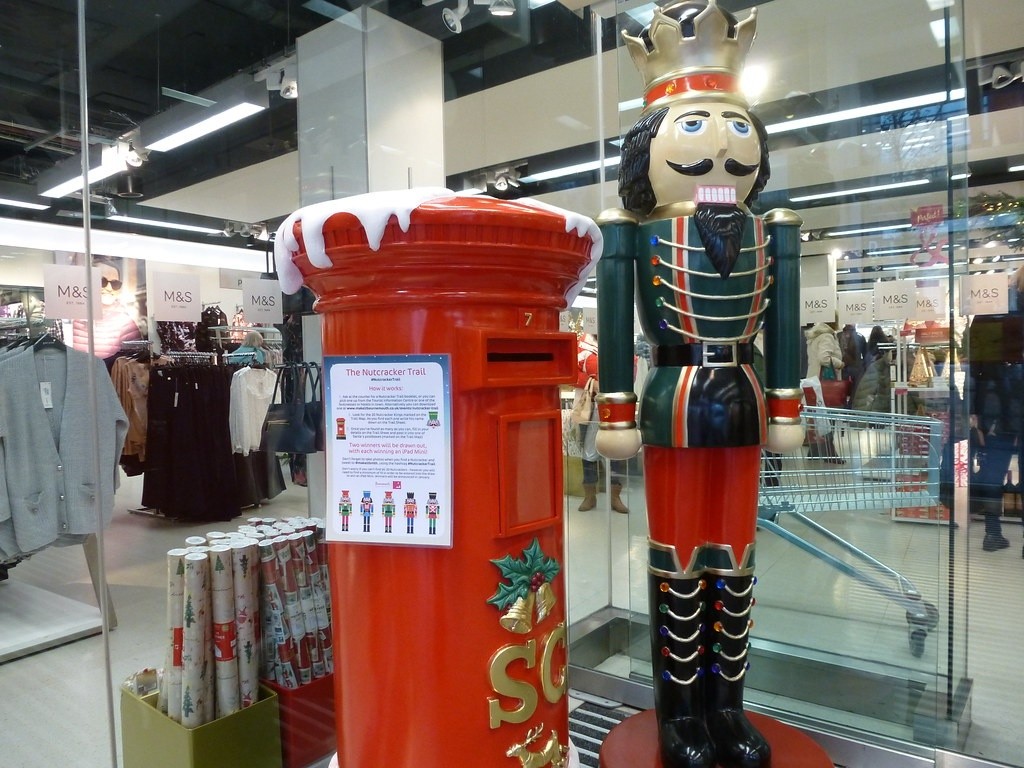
[102,277,122,291]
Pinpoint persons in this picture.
[569,333,638,513]
[598,0,803,768]
[229,330,265,365]
[864,326,889,371]
[968,265,1024,559]
[73,259,141,359]
[804,310,846,464]
[837,324,867,382]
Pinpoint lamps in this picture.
[224,221,252,238]
[440,0,470,34]
[446,173,488,196]
[138,13,270,154]
[488,0,517,16]
[513,139,621,183]
[991,64,1015,89]
[124,140,149,168]
[494,159,528,191]
[36,140,128,199]
[280,63,299,99]
[104,200,225,234]
[0,180,52,211]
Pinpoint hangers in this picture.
[879,343,947,361]
[0,317,66,354]
[112,339,283,376]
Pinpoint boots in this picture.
[578,483,597,511]
[611,483,628,513]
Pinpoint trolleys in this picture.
[755,398,948,660]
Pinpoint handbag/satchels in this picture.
[258,361,323,453]
[570,378,596,425]
[803,356,849,408]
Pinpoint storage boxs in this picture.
[257,674,336,768]
[119,668,283,767]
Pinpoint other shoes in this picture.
[807,450,825,460]
[802,431,825,445]
[824,452,846,464]
[982,535,1009,550]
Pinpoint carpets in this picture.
[569,702,636,768]
